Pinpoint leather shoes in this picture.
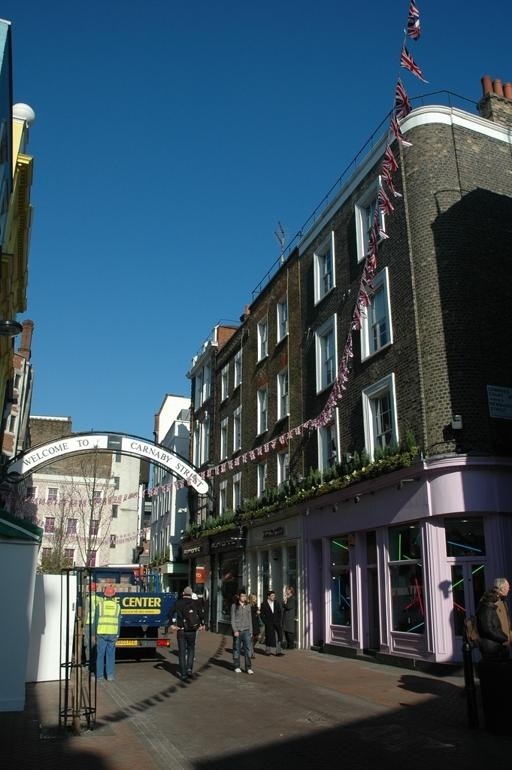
[266,653,285,656]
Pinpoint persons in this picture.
[91,584,121,682]
[476,578,511,710]
[82,582,103,667]
[168,585,206,680]
[230,584,297,674]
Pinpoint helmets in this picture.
[89,583,97,591]
[104,587,115,597]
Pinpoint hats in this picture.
[184,587,192,595]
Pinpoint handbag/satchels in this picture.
[465,613,482,649]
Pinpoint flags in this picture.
[6,420,312,506]
[312,1,429,437]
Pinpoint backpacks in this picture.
[183,606,201,631]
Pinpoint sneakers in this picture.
[247,669,254,675]
[97,678,114,681]
[182,671,192,679]
[234,667,241,673]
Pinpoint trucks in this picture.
[75,568,178,660]
